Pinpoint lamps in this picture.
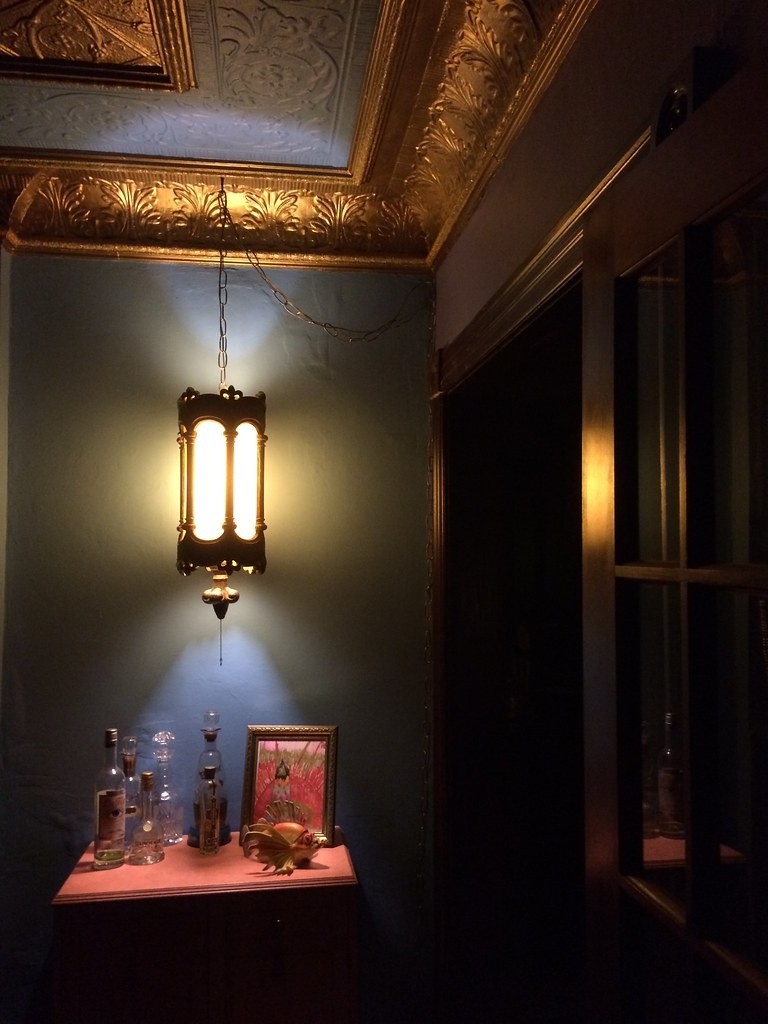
[176,174,267,665]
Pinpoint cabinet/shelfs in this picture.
[51,835,360,1024]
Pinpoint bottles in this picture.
[93,710,232,868]
[654,711,690,838]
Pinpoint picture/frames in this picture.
[240,724,339,849]
